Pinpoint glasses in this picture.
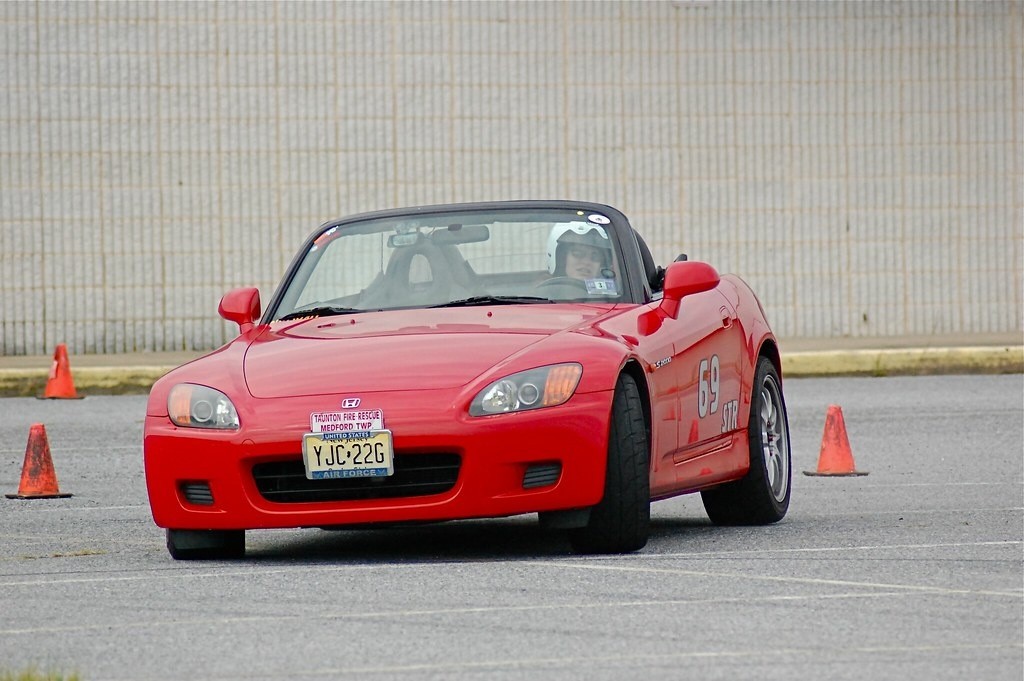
[567,248,604,262]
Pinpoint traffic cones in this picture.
[803,405,869,477]
[5,422,74,501]
[37,343,85,400]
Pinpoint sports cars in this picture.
[142,200,791,557]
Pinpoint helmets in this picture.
[548,221,611,278]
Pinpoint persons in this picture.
[546,220,613,280]
